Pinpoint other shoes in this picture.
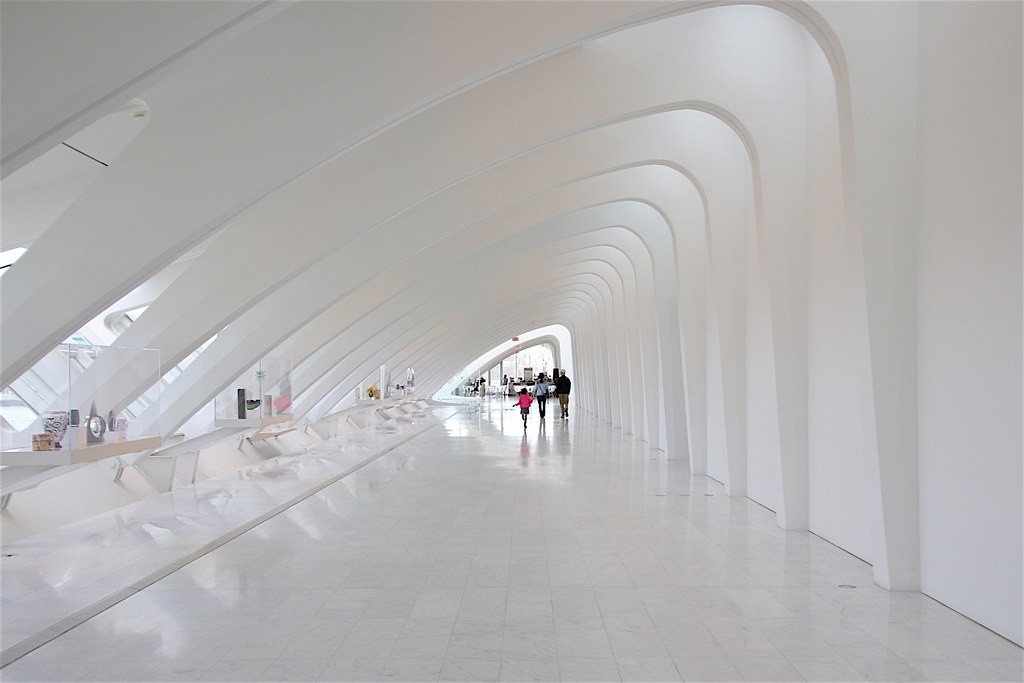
[566,411,569,417]
[561,415,564,418]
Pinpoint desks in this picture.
[513,386,557,399]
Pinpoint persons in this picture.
[465,379,476,394]
[512,388,533,428]
[519,428,530,467]
[554,369,571,418]
[533,372,550,418]
[556,418,571,466]
[537,418,550,463]
[502,374,508,396]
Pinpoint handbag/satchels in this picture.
[545,393,550,399]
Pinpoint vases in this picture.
[42,408,68,446]
